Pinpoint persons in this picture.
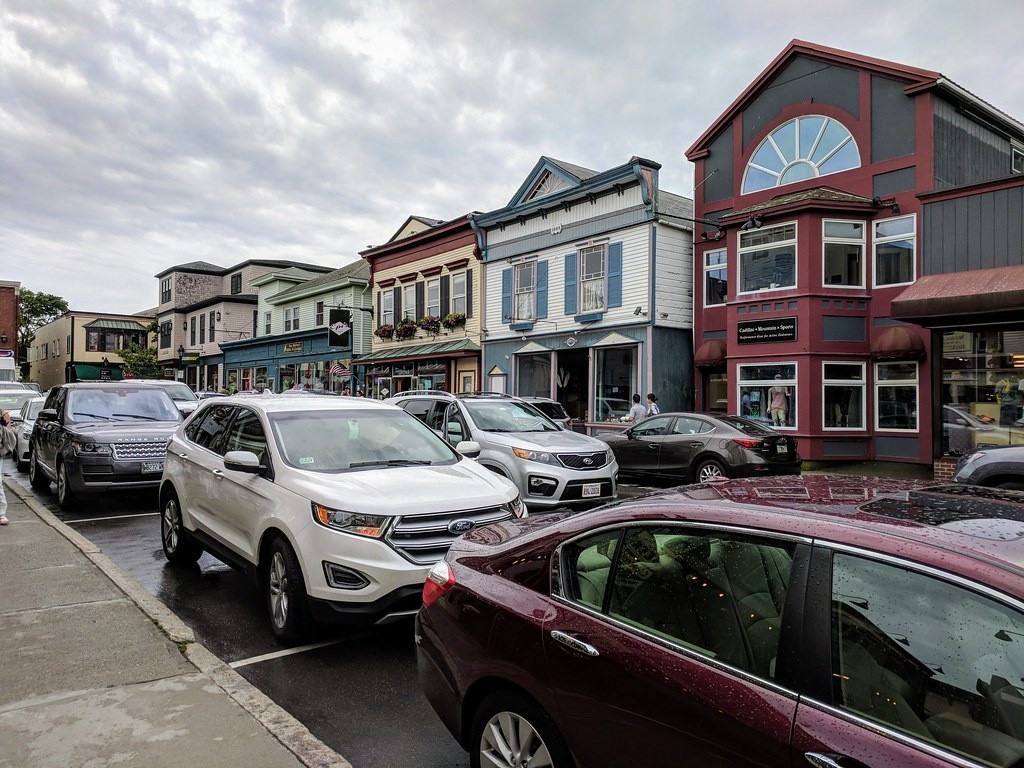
[340,386,365,397]
[289,380,310,390]
[742,390,753,416]
[207,385,275,394]
[624,393,660,425]
[767,374,791,427]
[956,375,968,404]
[556,368,570,410]
[995,374,1024,428]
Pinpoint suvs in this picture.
[378,389,620,512]
[27,384,185,510]
[160,390,533,649]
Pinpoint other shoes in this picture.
[0,517,9,524]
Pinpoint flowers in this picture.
[370,312,465,342]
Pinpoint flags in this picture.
[329,362,353,377]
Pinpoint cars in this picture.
[0,380,50,429]
[592,412,803,484]
[6,397,48,471]
[120,377,229,420]
[413,471,1024,768]
[509,395,572,432]
[953,443,1024,493]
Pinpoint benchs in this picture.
[575,540,915,713]
